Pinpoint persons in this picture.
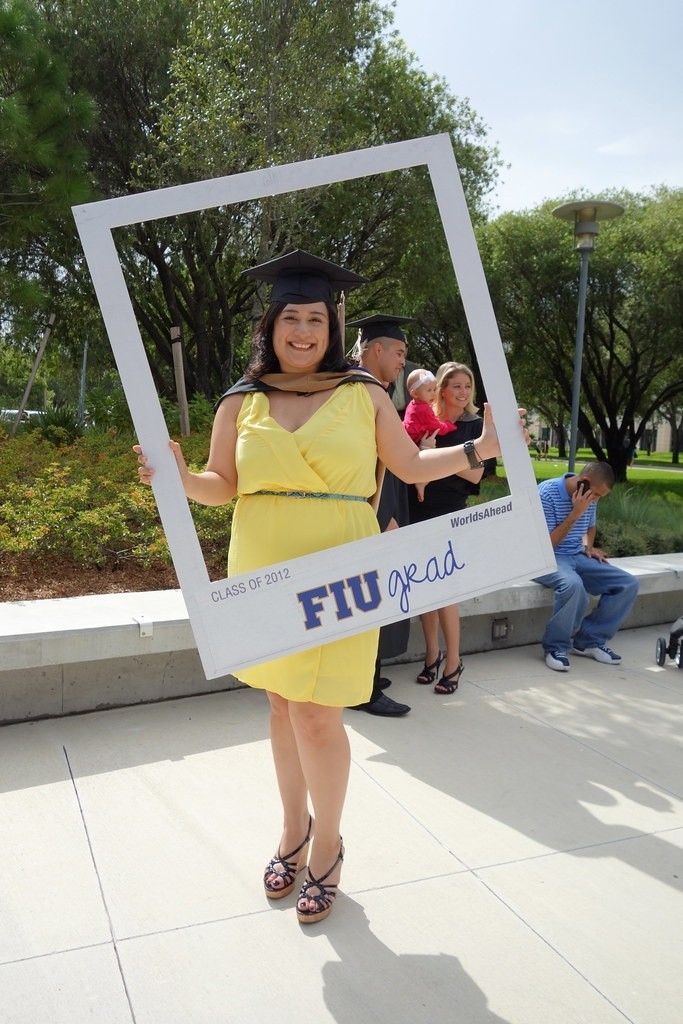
[532,462,639,670]
[535,437,549,461]
[133,249,531,922]
[629,447,638,467]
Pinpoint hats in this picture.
[344,313,417,359]
[240,249,370,358]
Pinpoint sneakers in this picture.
[573,644,621,665]
[544,651,570,671]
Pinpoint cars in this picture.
[74,410,112,430]
[1,410,47,424]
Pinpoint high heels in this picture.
[295,835,344,921]
[264,813,312,898]
[434,659,464,693]
[417,649,445,684]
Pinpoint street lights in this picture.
[550,199,626,479]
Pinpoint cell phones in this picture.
[577,481,590,496]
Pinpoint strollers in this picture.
[656,615,683,668]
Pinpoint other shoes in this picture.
[377,677,391,690]
[362,694,411,717]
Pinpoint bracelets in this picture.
[474,447,485,462]
[464,439,484,469]
